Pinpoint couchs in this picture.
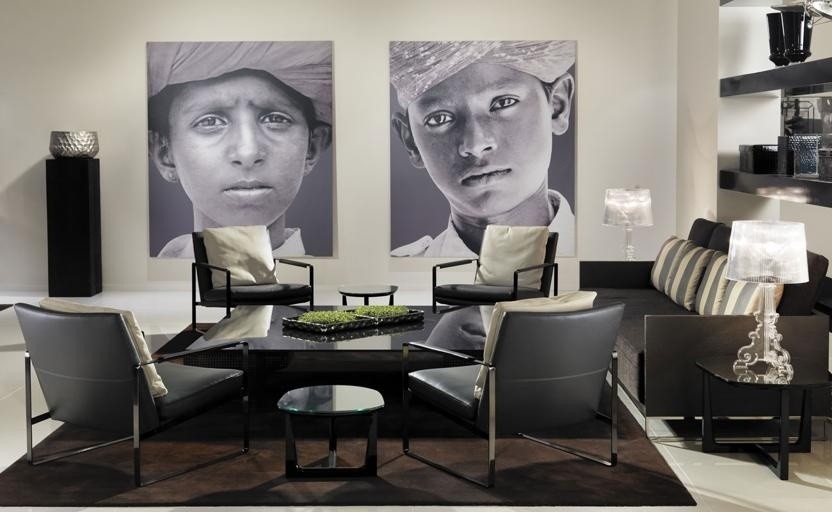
[579,218,832,442]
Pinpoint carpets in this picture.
[0,379,698,507]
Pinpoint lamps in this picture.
[725,220,810,378]
[602,187,655,262]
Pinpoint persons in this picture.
[390,40,576,259]
[148,43,332,257]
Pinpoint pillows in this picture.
[476,224,549,290]
[651,236,697,292]
[667,242,714,311]
[695,251,730,316]
[474,291,597,399]
[719,280,784,314]
[204,225,279,287]
[40,299,168,398]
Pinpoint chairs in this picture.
[192,232,314,328]
[402,302,626,490]
[14,302,249,487]
[432,232,558,316]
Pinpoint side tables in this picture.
[695,356,831,479]
[278,385,386,481]
[338,285,398,306]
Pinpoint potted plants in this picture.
[283,310,375,333]
[343,305,424,327]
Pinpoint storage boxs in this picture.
[739,144,778,174]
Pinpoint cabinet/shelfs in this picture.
[718,0,831,209]
